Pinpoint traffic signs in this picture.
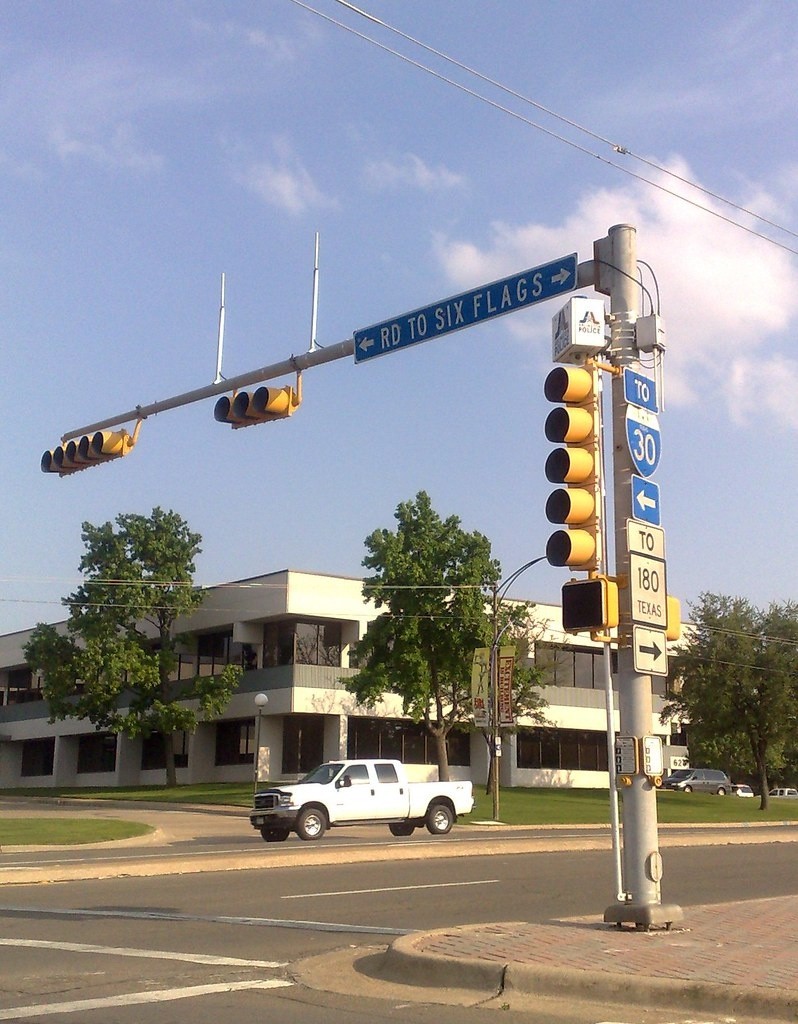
[352,251,580,365]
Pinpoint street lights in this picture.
[252,692,268,794]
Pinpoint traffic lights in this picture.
[39,427,133,479]
[543,361,600,570]
[212,384,294,430]
[561,577,619,639]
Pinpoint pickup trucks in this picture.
[249,758,475,844]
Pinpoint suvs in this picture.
[662,768,733,795]
[731,784,754,797]
[757,788,798,798]
